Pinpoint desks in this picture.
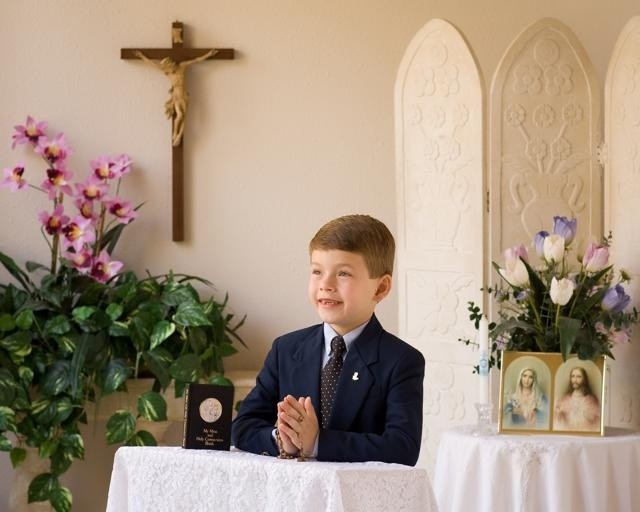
[438,421,639,509]
[112,438,431,512]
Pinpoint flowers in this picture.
[461,209,638,376]
[0,113,258,510]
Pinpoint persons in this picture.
[135,49,219,146]
[557,367,599,430]
[504,367,548,428]
[231,214,425,468]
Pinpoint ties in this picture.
[320,336,347,431]
